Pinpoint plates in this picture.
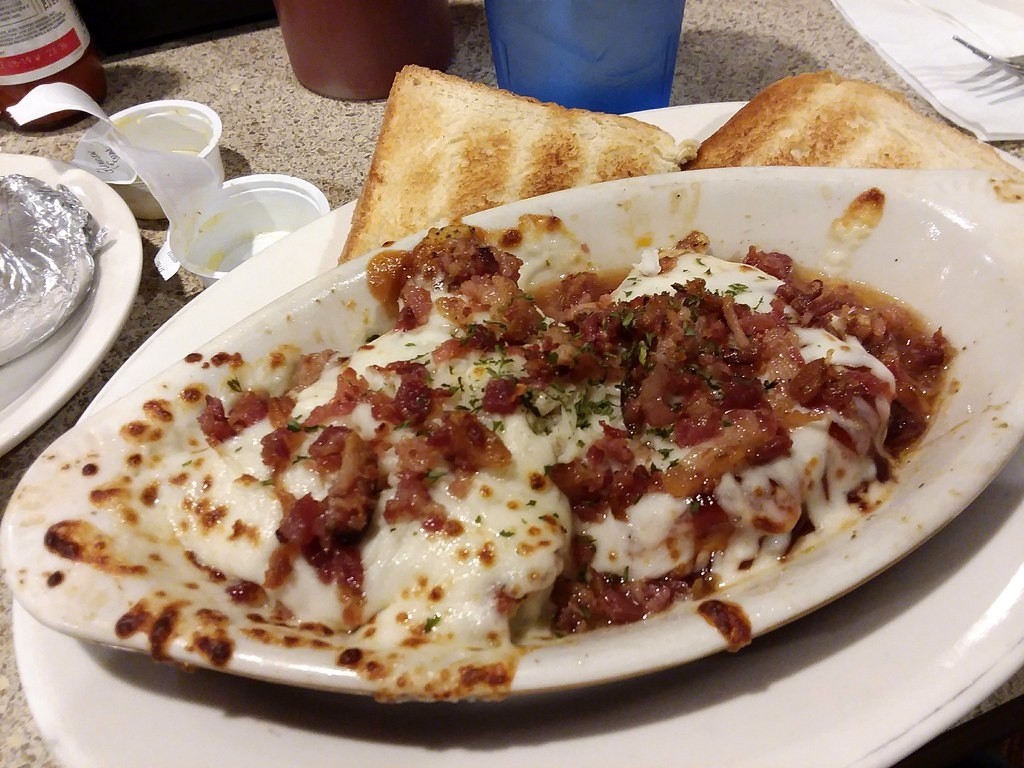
[0,102,1024,768]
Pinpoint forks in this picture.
[953,35,1024,77]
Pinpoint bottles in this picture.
[0,0,108,133]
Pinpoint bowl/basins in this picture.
[167,173,330,287]
[76,100,222,219]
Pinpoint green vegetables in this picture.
[223,263,770,487]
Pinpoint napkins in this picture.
[833,0,1024,141]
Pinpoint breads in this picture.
[677,70,1024,205]
[335,66,701,263]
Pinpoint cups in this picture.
[274,0,452,101]
[485,0,686,115]
